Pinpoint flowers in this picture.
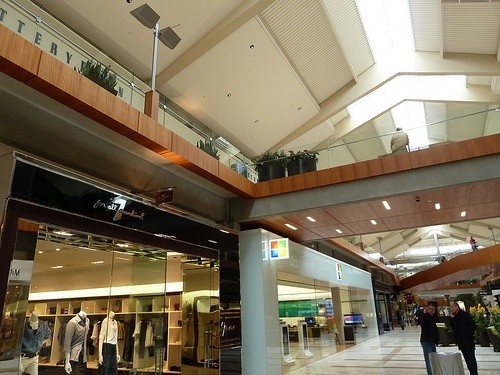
[469,303,500,328]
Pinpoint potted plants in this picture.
[197,140,220,160]
[81,59,119,96]
[255,148,320,182]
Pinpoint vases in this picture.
[485,327,500,352]
[476,328,490,347]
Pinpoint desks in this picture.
[289,328,298,342]
[308,327,329,338]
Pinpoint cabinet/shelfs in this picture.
[26,294,183,373]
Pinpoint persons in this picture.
[449,301,478,375]
[17,310,51,375]
[397,311,406,330]
[98,311,121,375]
[470,236,478,252]
[391,128,409,154]
[415,301,440,375]
[63,311,89,375]
